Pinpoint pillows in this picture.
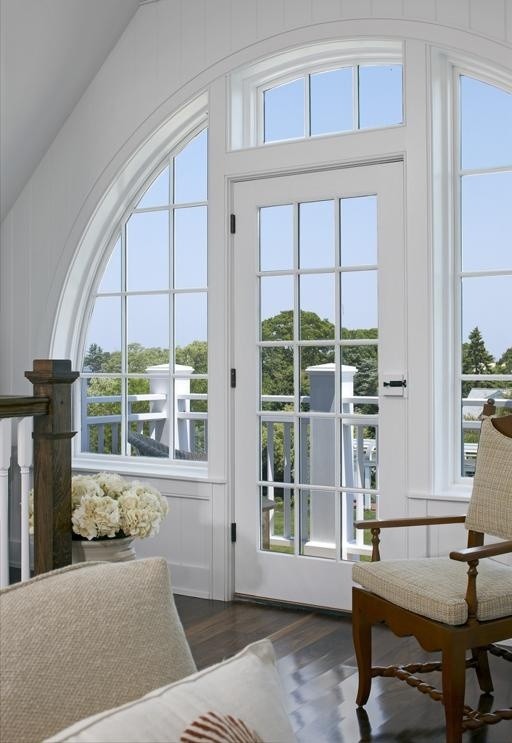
[45,638,303,742]
[1,558,200,743]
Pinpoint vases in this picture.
[71,536,137,564]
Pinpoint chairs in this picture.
[353,397,512,741]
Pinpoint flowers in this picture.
[19,470,170,540]
